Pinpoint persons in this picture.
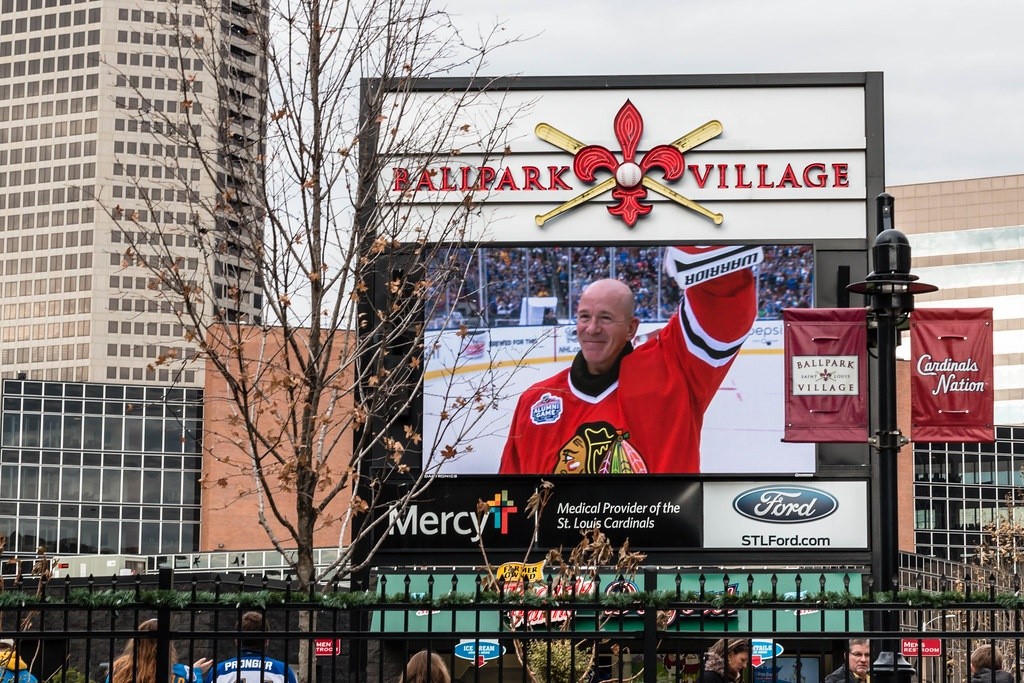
[697,637,752,683]
[206,611,298,683]
[0,638,38,683]
[399,649,452,683]
[422,244,814,329]
[104,619,214,683]
[499,244,763,475]
[825,637,876,683]
[970,644,1015,683]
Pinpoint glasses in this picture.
[849,652,870,659]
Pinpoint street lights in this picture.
[921,614,956,683]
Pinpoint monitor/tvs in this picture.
[517,296,558,326]
